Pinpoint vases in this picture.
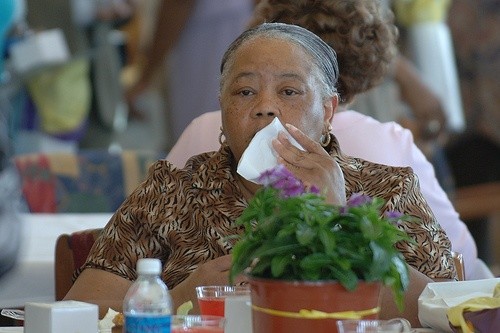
[240,276,382,333]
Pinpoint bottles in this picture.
[123,259,173,333]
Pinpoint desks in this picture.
[0,215,122,307]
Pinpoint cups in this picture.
[224,286,252,333]
[336,319,404,333]
[195,286,231,327]
[171,315,224,333]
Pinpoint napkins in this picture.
[237,118,305,186]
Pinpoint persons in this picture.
[65,22,457,327]
[166,0,499,280]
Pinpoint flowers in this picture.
[221,165,421,312]
